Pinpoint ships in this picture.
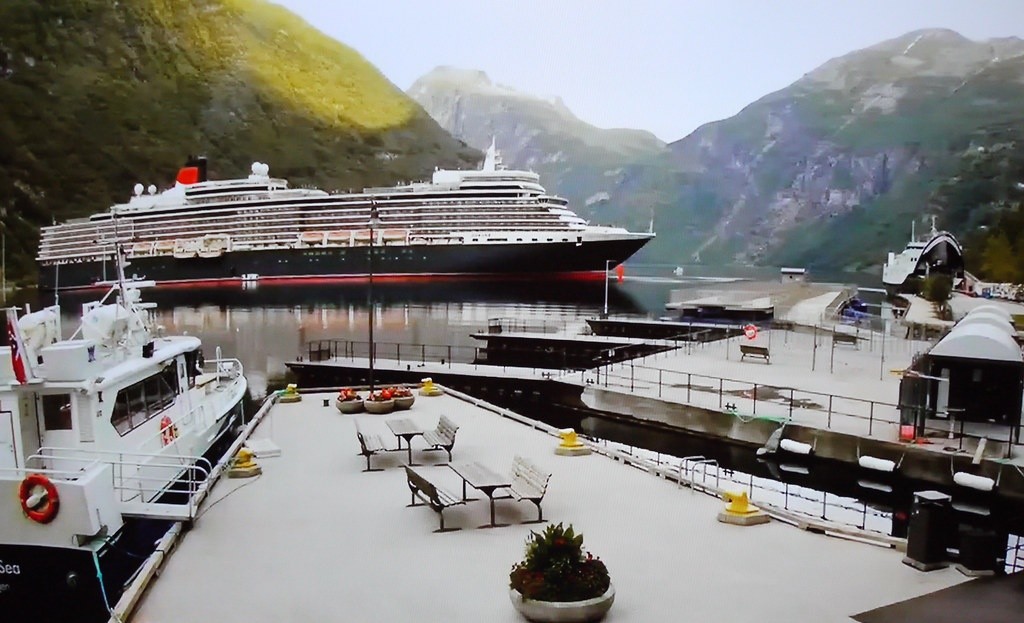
[880,214,967,299]
[34,139,657,308]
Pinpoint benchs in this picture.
[400,460,467,534]
[493,455,552,525]
[353,419,386,472]
[832,332,858,347]
[421,414,460,467]
[739,345,770,365]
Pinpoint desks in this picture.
[446,460,512,530]
[384,418,424,468]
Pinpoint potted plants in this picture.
[335,387,365,415]
[508,521,618,623]
[391,387,415,410]
[364,387,395,414]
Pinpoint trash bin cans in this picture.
[902,490,952,571]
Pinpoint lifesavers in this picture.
[161,416,174,445]
[21,475,59,524]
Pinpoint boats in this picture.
[466,317,700,370]
[665,282,780,316]
[0,240,248,601]
[578,299,1024,506]
[582,260,758,342]
[774,290,944,347]
[284,339,572,405]
[102,374,1023,623]
